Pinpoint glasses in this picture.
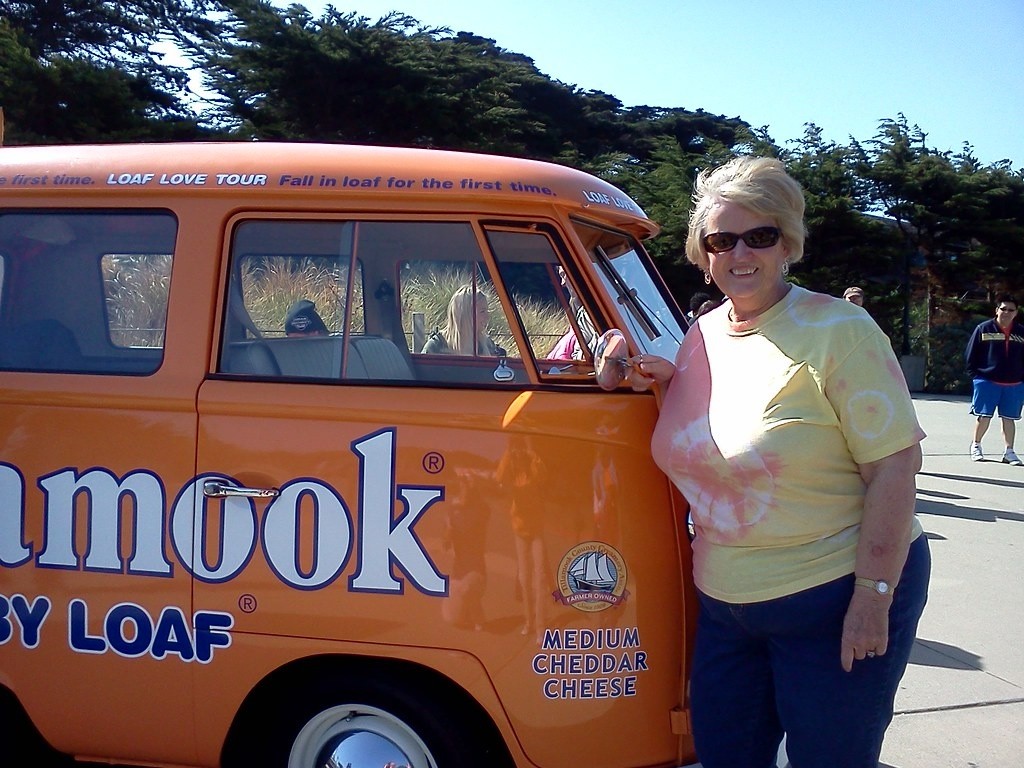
[703,225,785,254]
[999,307,1016,312]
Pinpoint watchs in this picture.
[855,577,894,596]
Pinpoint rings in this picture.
[637,355,643,362]
[625,376,628,380]
[866,651,876,657]
[877,649,886,656]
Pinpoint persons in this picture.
[546,329,577,360]
[557,265,601,360]
[622,156,931,768]
[843,287,864,306]
[285,300,331,337]
[963,295,1024,466]
[443,420,626,656]
[421,284,498,356]
[684,293,719,326]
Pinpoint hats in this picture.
[843,287,864,298]
[284,300,325,333]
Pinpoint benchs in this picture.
[227,336,417,376]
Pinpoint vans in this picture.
[0,140,701,767]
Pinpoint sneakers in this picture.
[1002,449,1022,466]
[969,440,984,462]
